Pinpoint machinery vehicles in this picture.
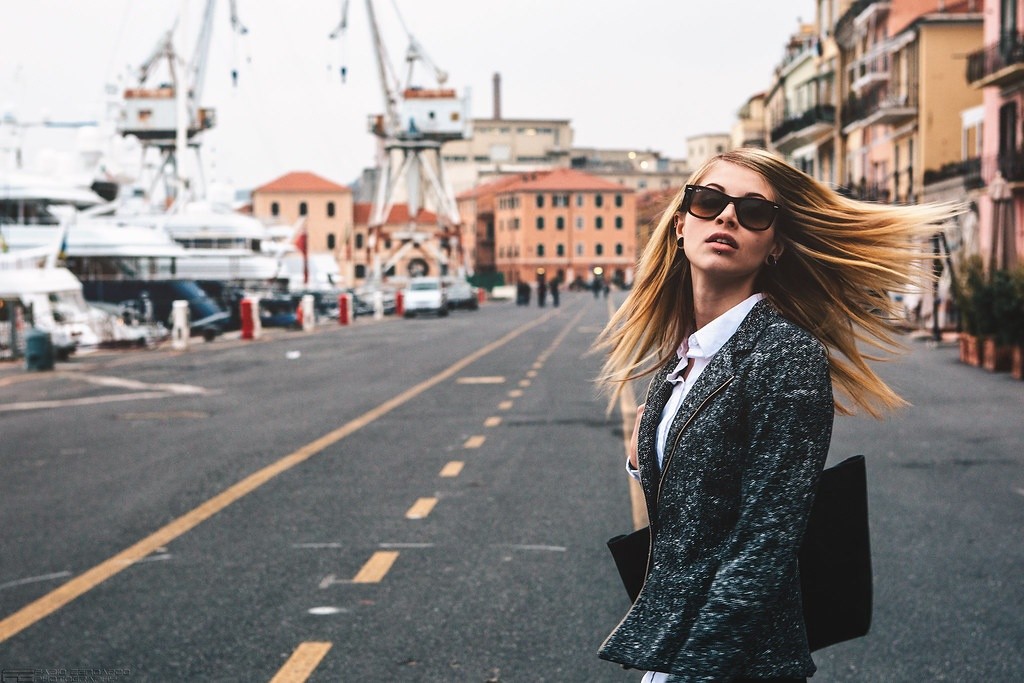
[86,0,250,213]
[321,0,480,293]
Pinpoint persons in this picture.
[577,148,969,683]
[515,274,610,307]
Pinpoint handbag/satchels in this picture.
[607,454,873,653]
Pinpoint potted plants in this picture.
[992,274,1024,380]
[968,263,1011,372]
[948,255,985,366]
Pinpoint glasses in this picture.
[680,185,782,231]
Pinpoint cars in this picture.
[401,274,477,317]
